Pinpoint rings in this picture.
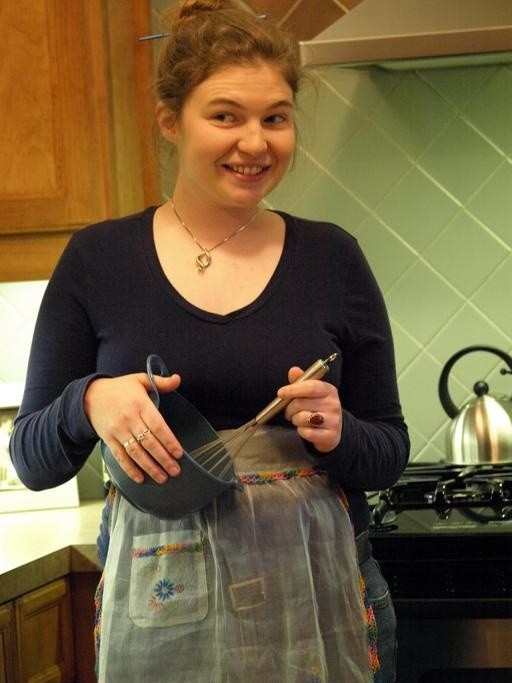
[123,438,135,448]
[306,411,325,428]
[134,428,150,442]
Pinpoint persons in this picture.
[8,0,415,683]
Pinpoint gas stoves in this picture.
[360,464,512,600]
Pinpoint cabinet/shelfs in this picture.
[4,572,75,680]
[3,0,161,280]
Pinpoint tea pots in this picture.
[437,342,512,466]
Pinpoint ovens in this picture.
[366,598,511,683]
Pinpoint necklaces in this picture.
[170,195,261,274]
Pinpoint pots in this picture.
[98,353,245,524]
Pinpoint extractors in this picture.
[297,0,512,76]
